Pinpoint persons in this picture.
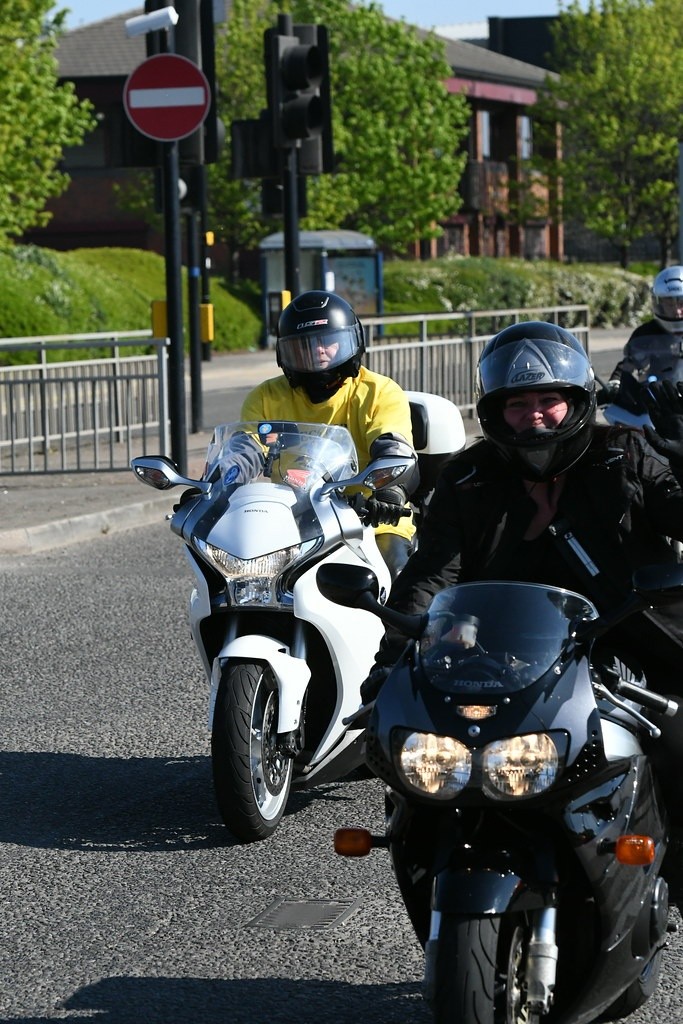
[601,266,683,402]
[359,320,683,831]
[175,291,417,589]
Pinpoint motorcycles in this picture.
[313,559,683,1022]
[130,387,467,837]
[591,333,683,440]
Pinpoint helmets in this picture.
[652,265,683,334]
[476,319,599,484]
[275,290,368,389]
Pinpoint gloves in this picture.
[358,660,395,707]
[364,486,407,529]
[643,379,683,492]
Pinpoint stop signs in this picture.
[124,52,211,144]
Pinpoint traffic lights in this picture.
[262,31,325,155]
